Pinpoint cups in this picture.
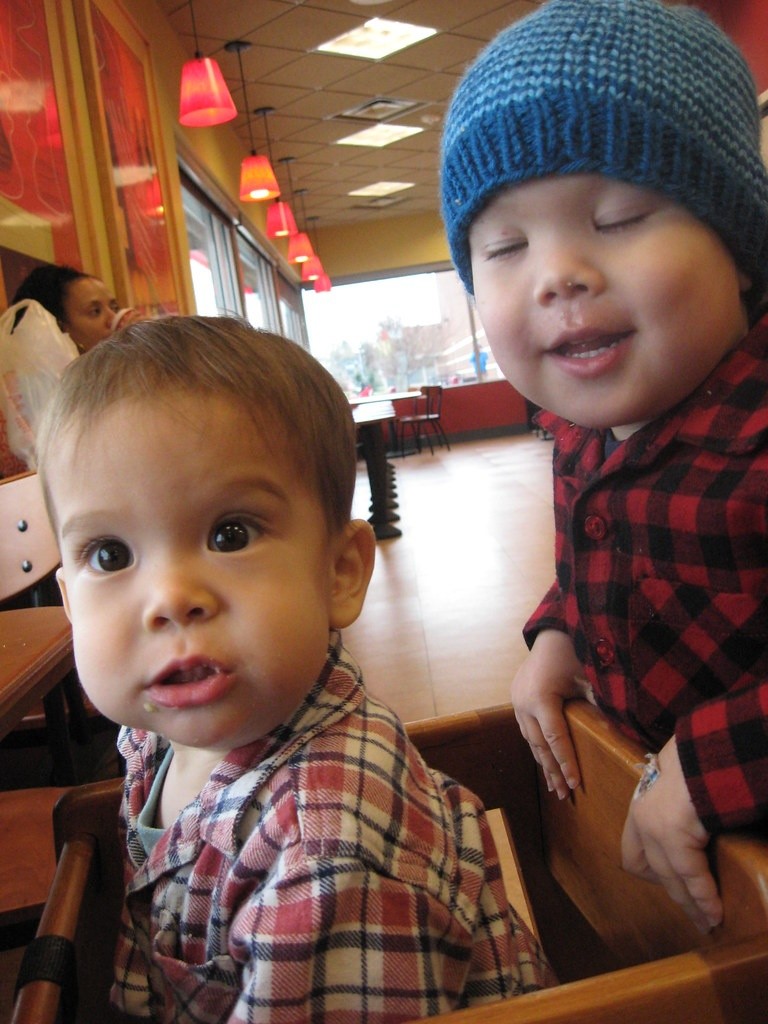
[109,306,139,330]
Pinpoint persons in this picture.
[438,0,768,933]
[34,315,555,1024]
[12,265,121,357]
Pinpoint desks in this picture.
[349,389,424,541]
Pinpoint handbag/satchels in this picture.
[0,299,80,472]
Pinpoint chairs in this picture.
[396,385,450,456]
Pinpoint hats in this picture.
[439,0,768,315]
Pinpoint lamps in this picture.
[179,0,332,294]
